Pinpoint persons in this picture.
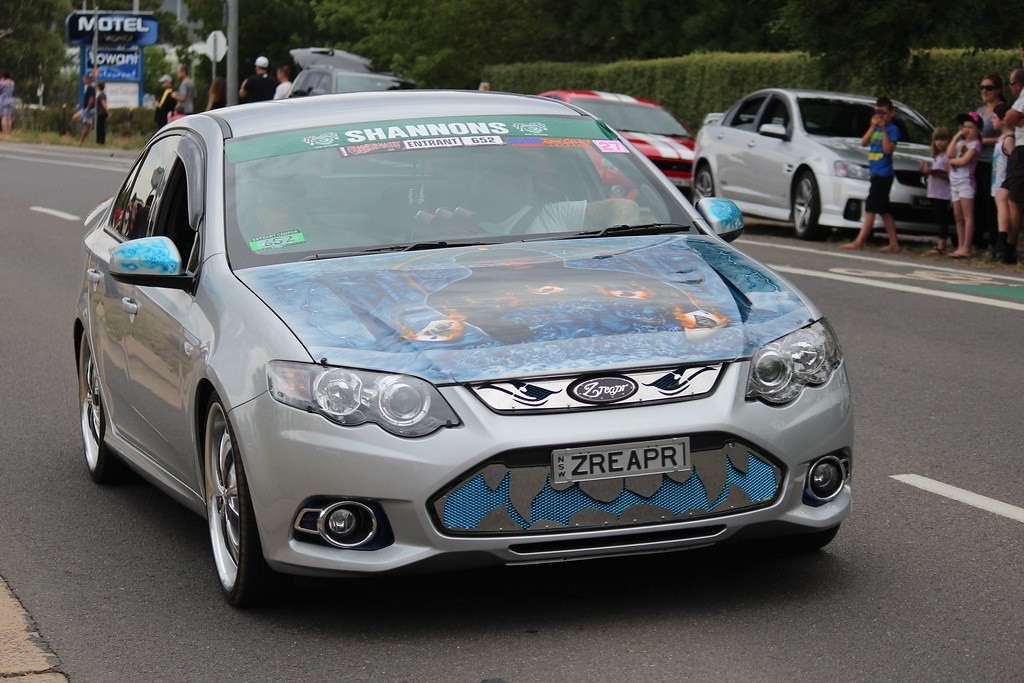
[206,76,226,111]
[919,112,983,257]
[167,66,197,123]
[240,56,276,104]
[273,65,293,100]
[72,75,96,144]
[0,69,15,139]
[975,69,1024,264]
[96,82,111,144]
[151,74,177,130]
[124,193,154,241]
[453,150,641,236]
[840,97,900,254]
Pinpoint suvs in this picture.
[282,47,418,99]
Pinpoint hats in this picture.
[255,56,268,67]
[159,74,174,83]
[957,111,984,131]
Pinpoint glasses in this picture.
[979,86,997,91]
[1009,81,1019,86]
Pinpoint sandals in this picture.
[929,246,945,255]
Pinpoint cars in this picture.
[73,91,852,611]
[539,90,697,200]
[692,87,955,242]
